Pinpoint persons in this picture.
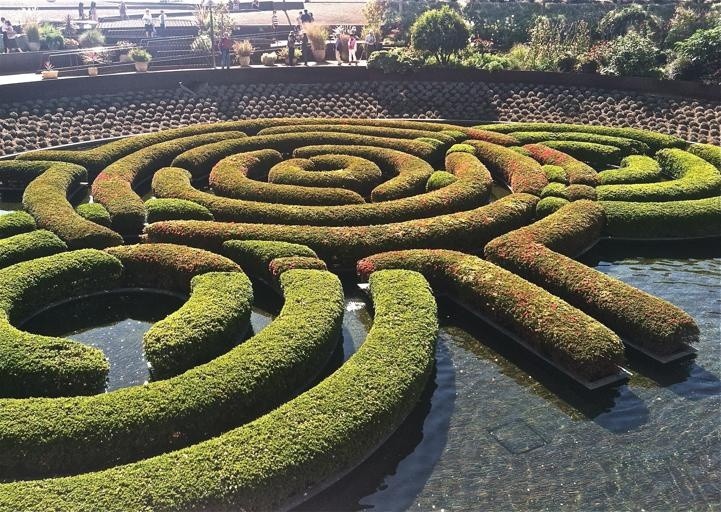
[273,9,377,67]
[218,33,233,70]
[5,20,23,53]
[159,10,167,32]
[2,17,8,55]
[142,9,154,38]
[79,1,127,30]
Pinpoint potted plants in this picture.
[40,23,151,81]
[238,22,350,67]
